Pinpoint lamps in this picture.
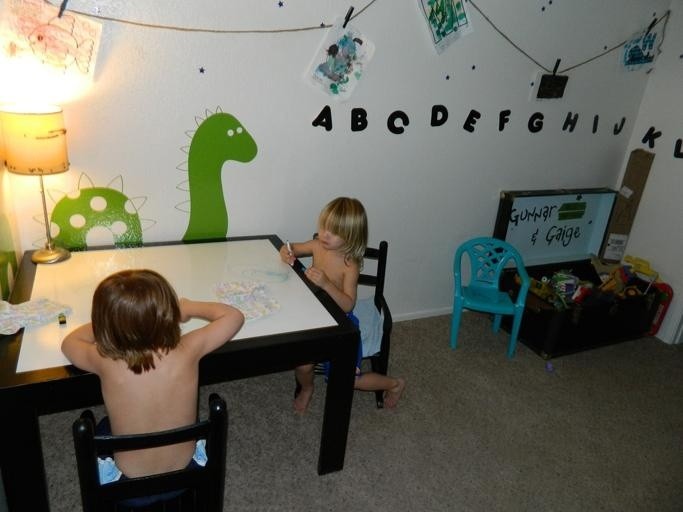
[2,108,74,264]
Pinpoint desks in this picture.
[1,236,363,511]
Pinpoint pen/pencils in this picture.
[286,239,295,267]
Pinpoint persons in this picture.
[60,269,246,481]
[278,196,407,418]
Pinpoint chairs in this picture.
[294,235,393,410]
[448,236,534,359]
[73,394,229,511]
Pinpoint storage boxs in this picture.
[487,187,669,361]
